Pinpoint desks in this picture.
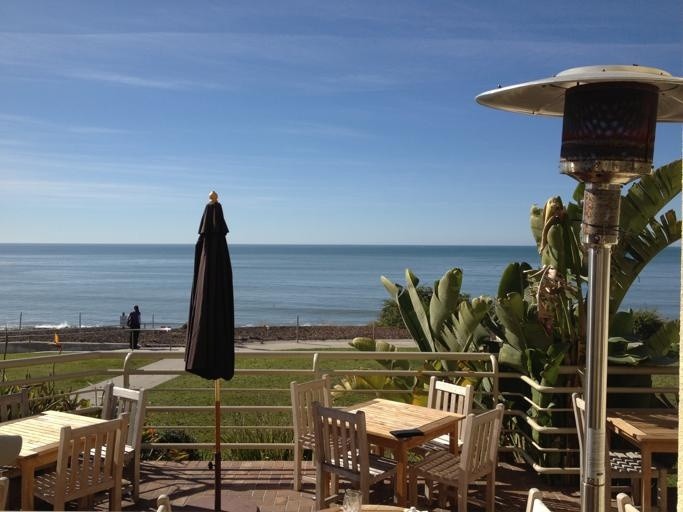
[608,407,678,512]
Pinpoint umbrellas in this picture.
[185,190,235,512]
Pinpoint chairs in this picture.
[0,382,172,511]
[289,374,505,511]
[526,486,643,512]
[571,392,660,512]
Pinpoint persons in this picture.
[120,312,127,324]
[127,305,141,350]
